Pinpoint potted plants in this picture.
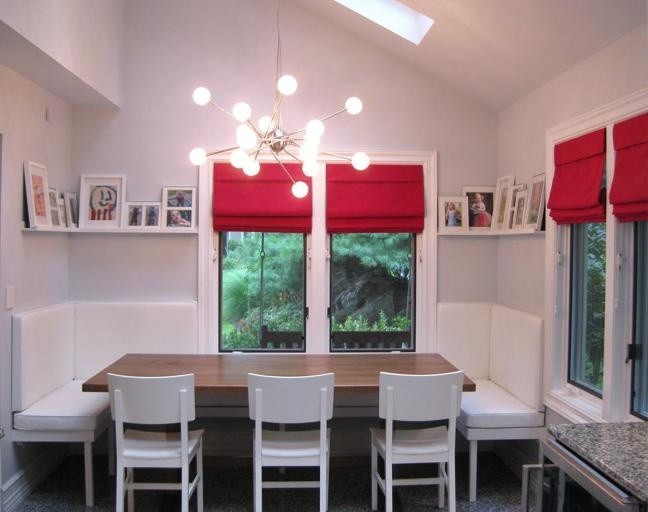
[162,186,197,231]
[48,187,62,227]
[56,198,68,227]
[23,160,53,229]
[505,183,527,233]
[529,171,546,232]
[489,174,516,233]
[438,196,470,233]
[127,201,162,228]
[79,174,127,228]
[512,189,529,233]
[461,185,496,232]
[63,191,79,227]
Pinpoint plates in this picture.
[520,421,623,512]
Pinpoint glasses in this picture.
[189,0,371,198]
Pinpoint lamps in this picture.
[248,371,336,512]
[106,372,206,512]
[369,369,465,512]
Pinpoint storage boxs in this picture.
[436,303,545,502]
[11,300,198,506]
